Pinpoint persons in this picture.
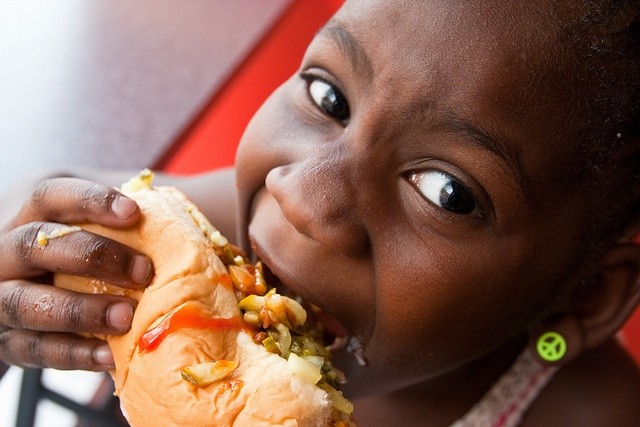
[0,0,639,426]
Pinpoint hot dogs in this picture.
[46,175,354,427]
